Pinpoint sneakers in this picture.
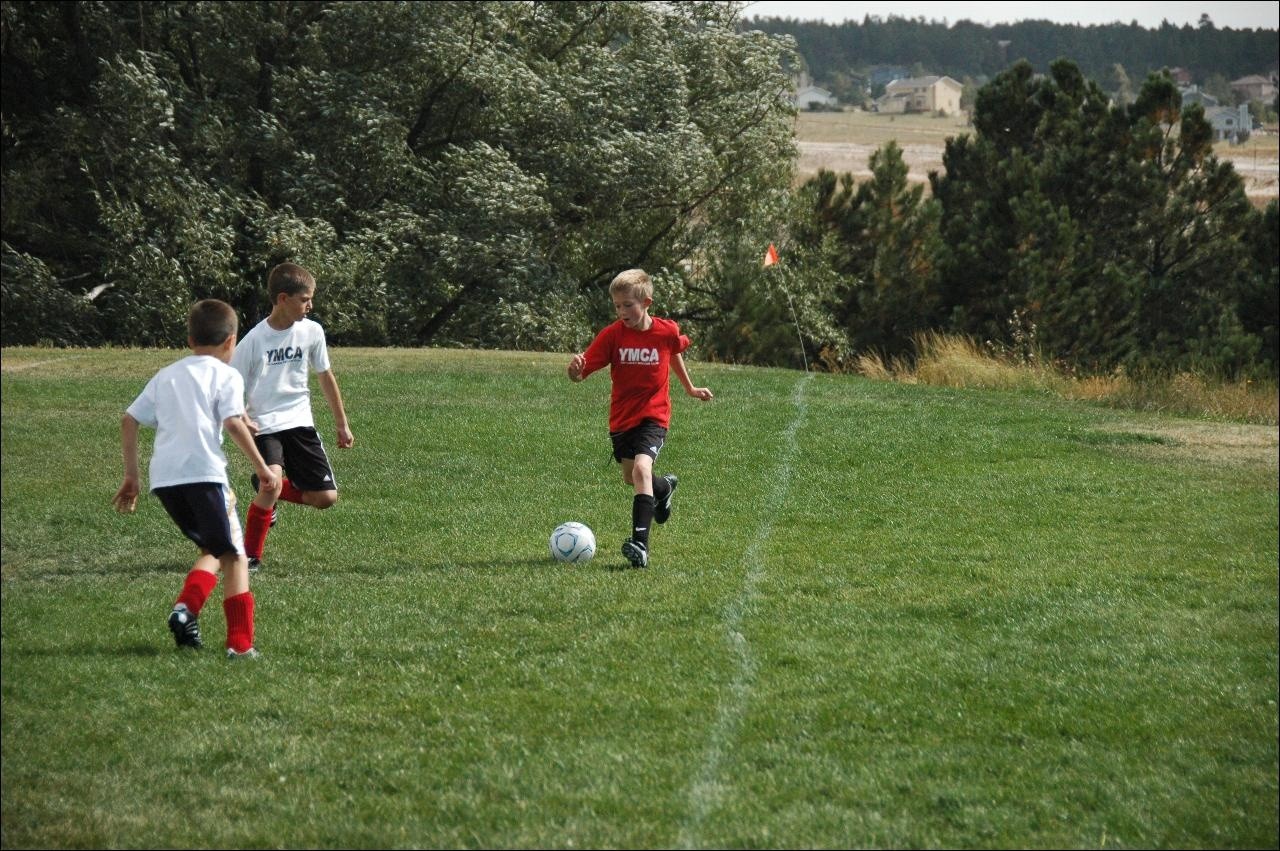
[168,603,203,649]
[246,557,260,572]
[620,536,648,568]
[250,473,277,527]
[653,473,678,524]
[225,648,259,660]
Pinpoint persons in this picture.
[112,297,278,661]
[568,268,713,570]
[226,263,355,574]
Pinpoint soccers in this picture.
[549,522,596,563]
